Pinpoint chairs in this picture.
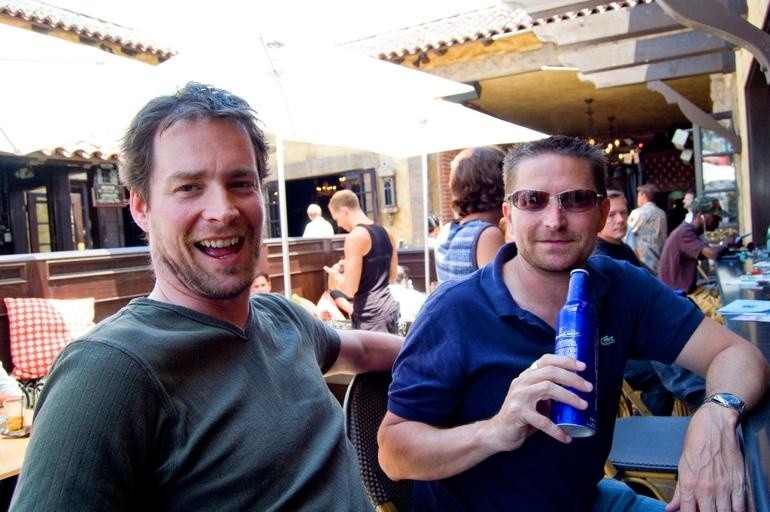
[601,377,694,504]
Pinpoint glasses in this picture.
[503,188,606,213]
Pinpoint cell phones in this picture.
[324,266,339,278]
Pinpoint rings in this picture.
[531,361,538,370]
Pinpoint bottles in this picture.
[554,268,596,437]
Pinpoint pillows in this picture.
[4,296,96,380]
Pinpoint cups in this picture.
[4,399,23,430]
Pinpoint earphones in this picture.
[701,215,704,220]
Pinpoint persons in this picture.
[7,81,410,510]
[592,182,736,497]
[378,135,770,512]
[323,189,437,334]
[302,204,335,237]
[435,146,507,288]
[251,271,271,296]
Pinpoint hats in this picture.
[687,196,728,219]
[635,182,661,193]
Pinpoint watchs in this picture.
[704,391,745,413]
